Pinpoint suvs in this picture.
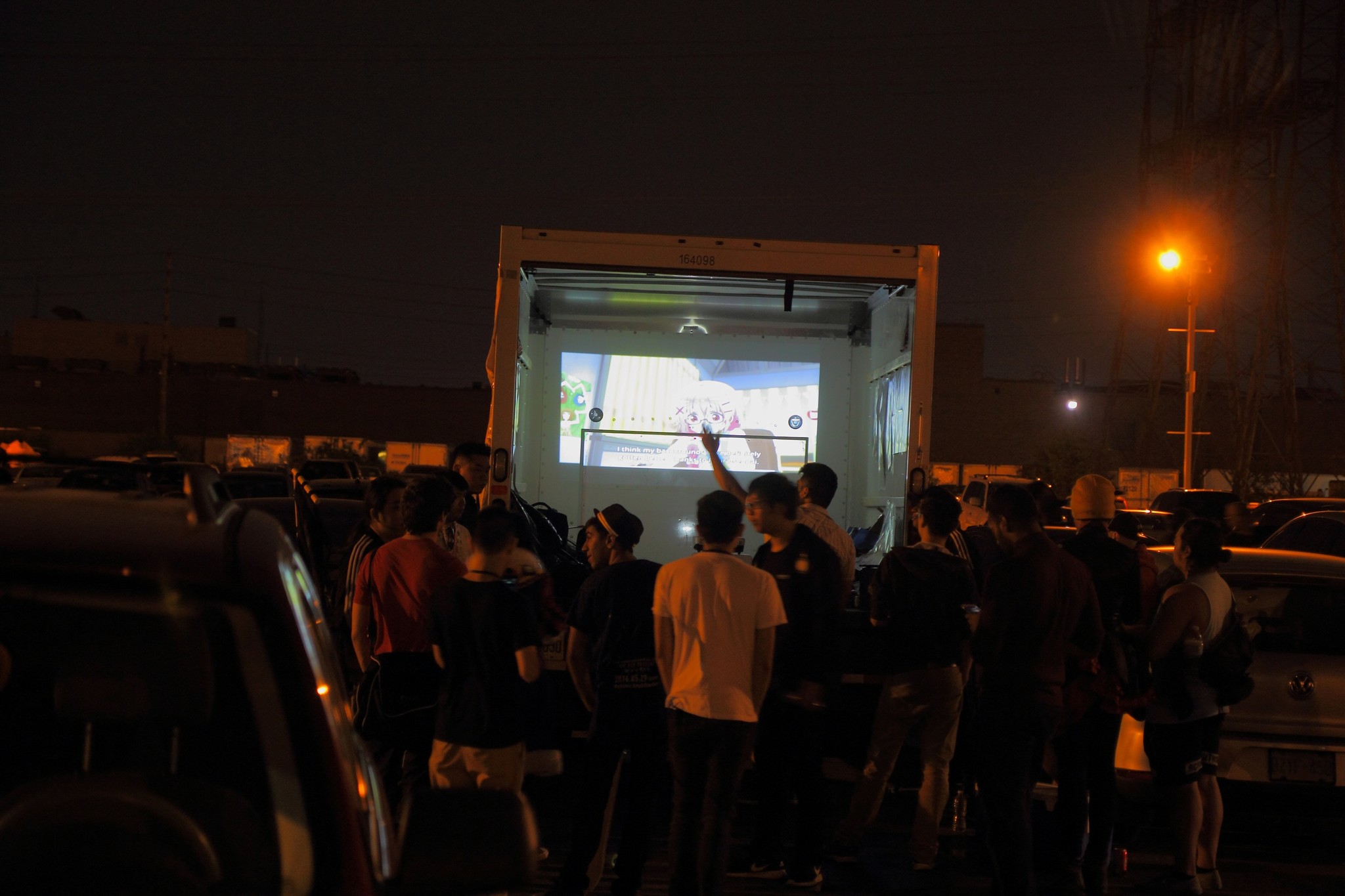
[0,455,449,896]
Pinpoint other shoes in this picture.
[545,886,587,896]
[725,858,787,878]
[1087,876,1108,896]
[1046,868,1085,888]
[908,842,939,868]
[1197,869,1223,890]
[835,834,862,862]
[786,866,825,886]
[1133,875,1201,896]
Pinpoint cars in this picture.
[954,473,1345,789]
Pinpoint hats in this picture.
[594,504,644,547]
[1070,474,1115,519]
[1109,513,1143,540]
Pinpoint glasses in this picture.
[744,500,767,509]
[911,513,922,527]
[446,526,455,551]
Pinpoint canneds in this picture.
[1113,847,1127,872]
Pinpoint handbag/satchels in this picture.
[1182,597,1256,707]
[531,502,568,544]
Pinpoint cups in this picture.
[962,605,981,636]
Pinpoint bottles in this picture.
[1185,625,1205,656]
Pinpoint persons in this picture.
[650,487,784,896]
[341,420,1235,896]
[423,496,543,895]
[838,493,978,872]
[1143,517,1236,896]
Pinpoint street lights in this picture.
[1156,247,1195,489]
